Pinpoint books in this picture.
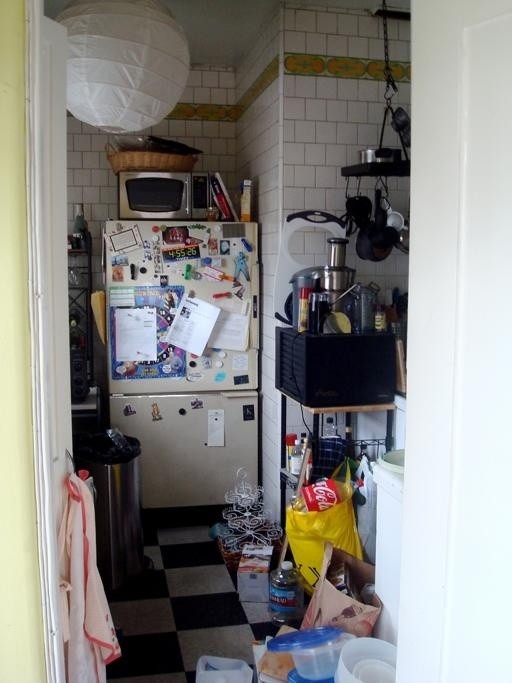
[209,180,226,219]
[214,171,240,221]
[211,175,232,221]
[239,178,253,220]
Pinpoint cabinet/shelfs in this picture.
[276,390,397,573]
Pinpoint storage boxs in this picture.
[237,543,274,602]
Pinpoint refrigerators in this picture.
[103,221,260,508]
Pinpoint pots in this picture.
[356,148,402,164]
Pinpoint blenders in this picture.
[292,237,356,329]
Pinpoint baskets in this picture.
[104,143,199,176]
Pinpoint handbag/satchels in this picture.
[286,455,383,637]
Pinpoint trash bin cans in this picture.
[81,435,141,595]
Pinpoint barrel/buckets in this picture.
[371,449,405,647]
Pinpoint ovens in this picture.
[274,326,397,407]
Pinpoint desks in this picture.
[68,232,102,435]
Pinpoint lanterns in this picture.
[52,0,191,136]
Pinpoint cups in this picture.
[309,293,329,335]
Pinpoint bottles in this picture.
[67,203,91,405]
[290,416,370,513]
[353,288,376,335]
[107,426,133,454]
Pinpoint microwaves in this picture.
[117,170,211,221]
